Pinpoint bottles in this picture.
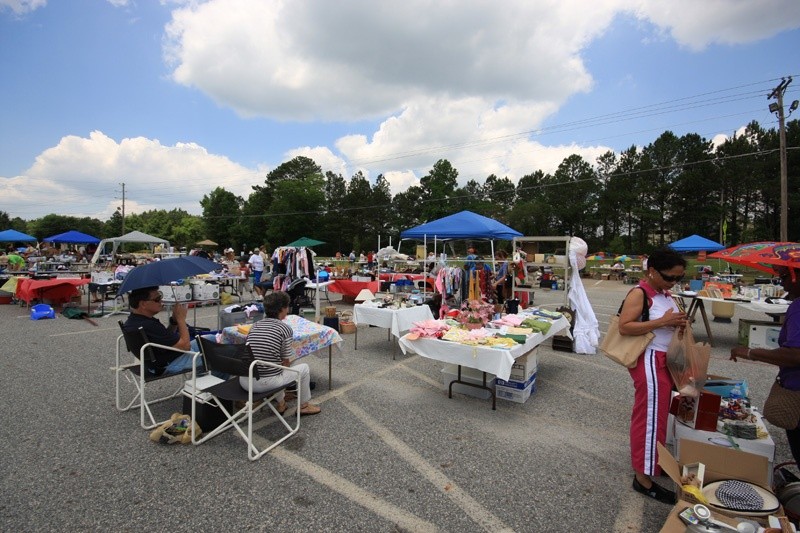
[728,383,742,399]
[390,284,397,294]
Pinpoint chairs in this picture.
[192,335,301,461]
[109,320,201,429]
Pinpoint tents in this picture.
[391,210,524,282]
[102,230,170,269]
[42,230,101,254]
[668,234,724,275]
[0,229,38,250]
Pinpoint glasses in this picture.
[657,268,684,283]
[142,294,162,303]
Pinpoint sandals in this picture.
[294,403,322,417]
[278,403,287,415]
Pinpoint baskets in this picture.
[339,309,356,334]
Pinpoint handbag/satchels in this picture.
[763,381,800,431]
[150,412,203,444]
[599,287,657,369]
[665,320,710,396]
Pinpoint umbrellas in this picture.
[196,240,219,246]
[116,255,223,307]
[584,254,604,266]
[284,237,326,247]
[616,255,633,265]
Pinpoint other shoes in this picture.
[631,474,677,504]
[255,295,264,301]
[659,468,668,475]
[234,399,258,408]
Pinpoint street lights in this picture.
[767,98,800,242]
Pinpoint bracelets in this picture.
[747,348,753,360]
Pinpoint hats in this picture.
[254,248,259,252]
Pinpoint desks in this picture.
[398,307,573,410]
[218,276,246,303]
[671,292,788,338]
[87,282,122,316]
[222,315,344,390]
[590,266,642,279]
[352,301,435,360]
[303,274,417,306]
[0,276,92,310]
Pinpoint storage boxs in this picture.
[656,375,785,533]
[159,283,219,301]
[324,307,354,334]
[441,365,496,399]
[182,376,233,433]
[495,344,541,403]
[739,319,782,349]
[94,272,115,283]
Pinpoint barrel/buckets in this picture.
[323,317,339,333]
[341,321,354,334]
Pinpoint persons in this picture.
[123,285,217,374]
[612,255,649,280]
[619,242,687,506]
[240,290,322,418]
[725,263,800,471]
[189,245,288,302]
[335,249,435,273]
[0,242,162,312]
[464,246,512,302]
